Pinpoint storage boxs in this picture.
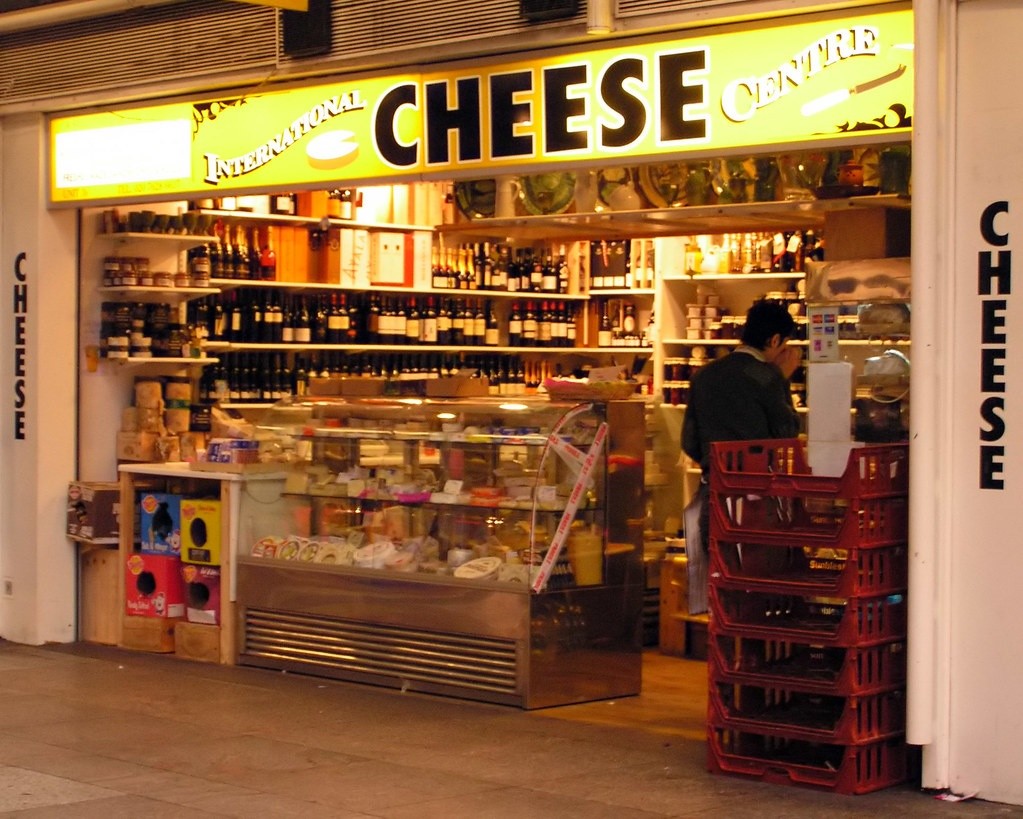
[340,378,387,396]
[307,377,342,395]
[122,492,221,627]
[703,437,923,795]
[65,481,120,545]
[424,367,491,398]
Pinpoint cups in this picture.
[686,294,722,340]
[590,329,612,346]
[103,209,212,236]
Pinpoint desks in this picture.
[117,461,312,666]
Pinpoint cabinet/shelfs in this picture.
[94,232,222,373]
[185,208,910,408]
[235,394,645,708]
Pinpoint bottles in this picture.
[432,241,569,294]
[187,287,590,403]
[211,220,277,281]
[599,302,655,347]
[199,188,352,219]
[684,229,825,275]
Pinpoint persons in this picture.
[680,299,801,556]
